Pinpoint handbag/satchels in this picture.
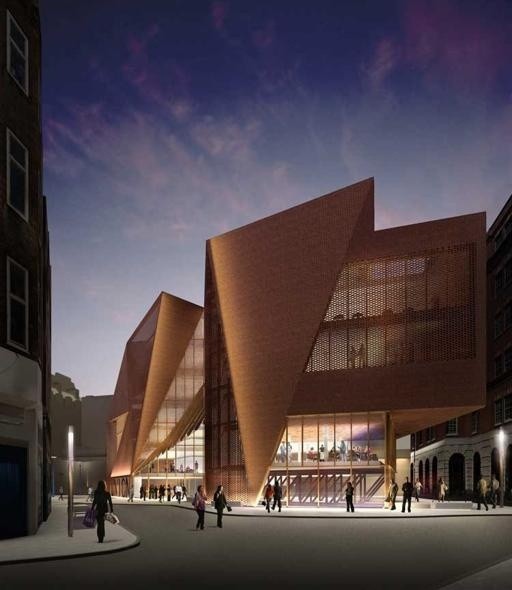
[84,508,95,527]
[227,506,232,511]
[262,501,266,505]
[105,513,119,524]
